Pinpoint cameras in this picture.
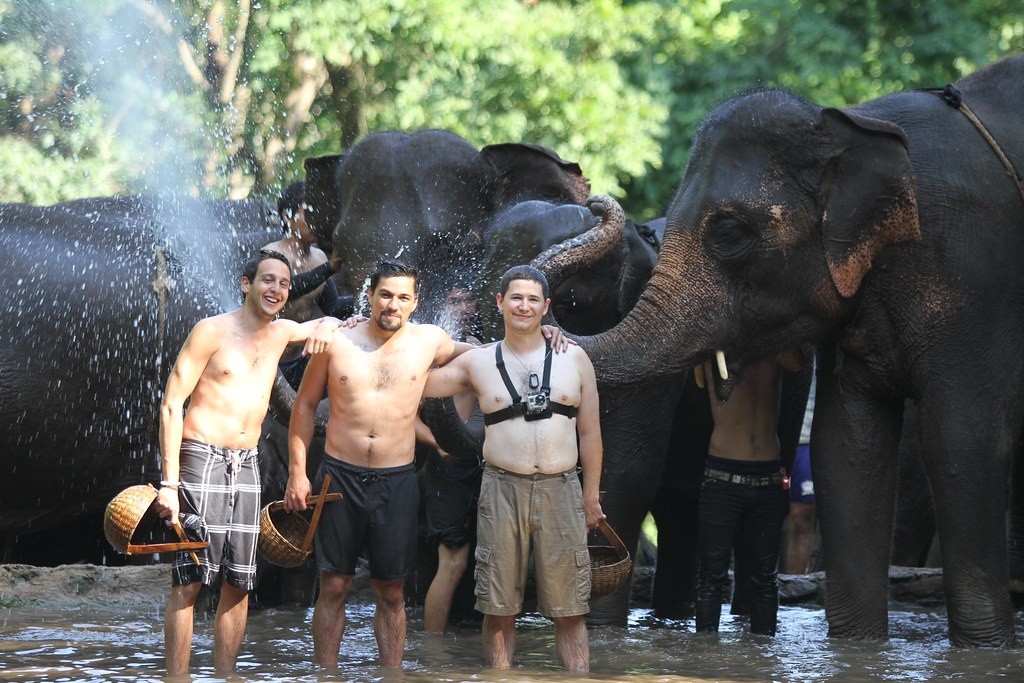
[526,392,546,413]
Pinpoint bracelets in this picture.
[160,481,182,490]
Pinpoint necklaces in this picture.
[504,342,555,389]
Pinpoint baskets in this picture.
[587,519,633,599]
[103,483,209,566]
[257,473,343,568]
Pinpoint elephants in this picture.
[1,55,1024,651]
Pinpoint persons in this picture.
[693,352,822,638]
[415,285,484,634]
[259,181,343,402]
[283,261,578,668]
[342,265,607,672]
[154,249,341,675]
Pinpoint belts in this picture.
[703,467,787,488]
